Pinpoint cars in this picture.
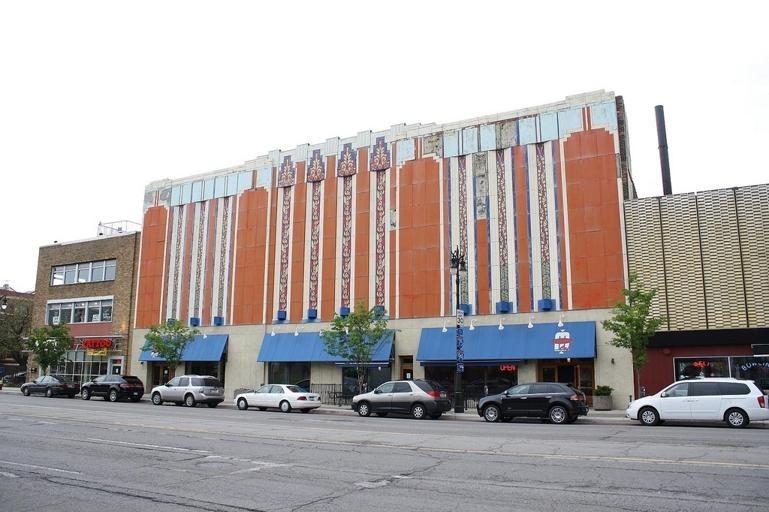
[20,374,79,398]
[233,383,322,413]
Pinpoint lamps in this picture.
[270,324,351,338]
[202,333,208,339]
[439,310,567,333]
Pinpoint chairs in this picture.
[328,390,355,406]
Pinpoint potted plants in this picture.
[590,385,616,410]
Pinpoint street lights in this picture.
[449,243,469,412]
[0,293,7,312]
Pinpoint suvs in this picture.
[351,379,452,421]
[477,382,591,424]
[150,374,225,409]
[81,375,144,403]
[625,377,769,427]
[465,376,515,397]
